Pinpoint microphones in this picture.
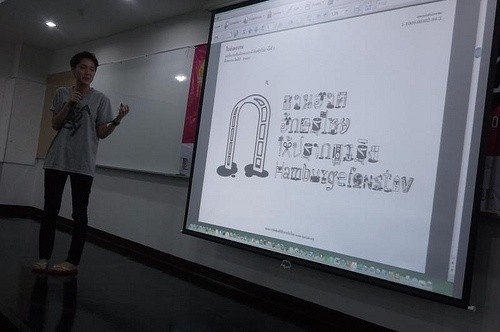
[68,92,81,121]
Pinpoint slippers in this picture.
[47,263,79,276]
[31,258,48,274]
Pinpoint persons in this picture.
[31,50,131,276]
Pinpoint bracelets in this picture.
[113,119,121,126]
